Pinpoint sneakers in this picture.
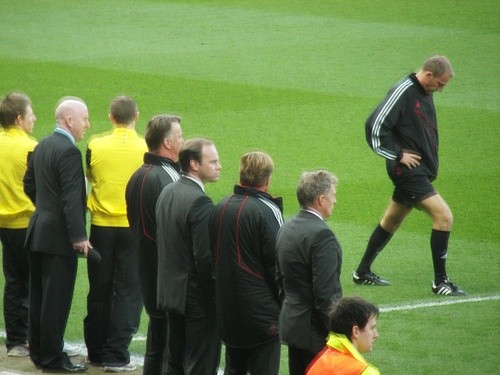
[431,275,467,296]
[353,270,391,286]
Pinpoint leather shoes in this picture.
[58,363,89,372]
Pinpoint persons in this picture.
[124,113,184,375]
[209,151,285,375]
[305,296,380,375]
[0,92,39,356]
[23,99,93,373]
[155,137,221,375]
[353,55,468,295]
[277,171,342,375]
[83,96,150,372]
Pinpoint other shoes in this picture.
[103,359,136,371]
[8,344,30,356]
[63,341,82,356]
